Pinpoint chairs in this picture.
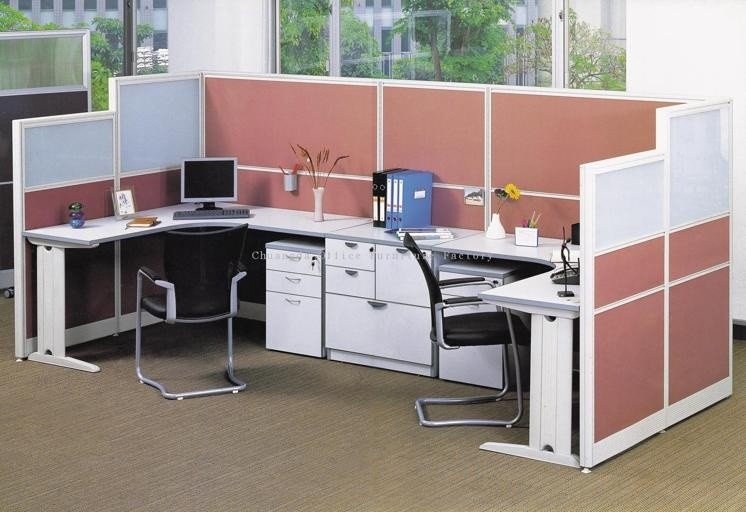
[135,222,250,402]
[402,232,531,430]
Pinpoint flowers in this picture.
[494,183,521,214]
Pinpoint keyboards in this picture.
[173,209,249,220]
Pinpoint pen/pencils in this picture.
[522,210,543,228]
[279,166,286,175]
[287,164,300,175]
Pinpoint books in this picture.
[124,217,157,231]
[396,225,454,240]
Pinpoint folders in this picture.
[396,233,452,237]
[384,227,448,233]
[392,170,425,229]
[379,169,409,227]
[387,169,412,227]
[373,168,401,227]
[398,172,432,228]
[400,236,453,241]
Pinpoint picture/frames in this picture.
[108,184,139,222]
[464,186,485,207]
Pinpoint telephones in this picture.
[550,265,580,285]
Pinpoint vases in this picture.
[485,213,506,239]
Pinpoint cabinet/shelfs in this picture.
[264,239,325,359]
[324,237,435,379]
[436,262,517,390]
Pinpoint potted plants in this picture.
[289,139,351,223]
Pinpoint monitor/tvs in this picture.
[180,157,237,211]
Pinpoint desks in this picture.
[21,202,580,469]
